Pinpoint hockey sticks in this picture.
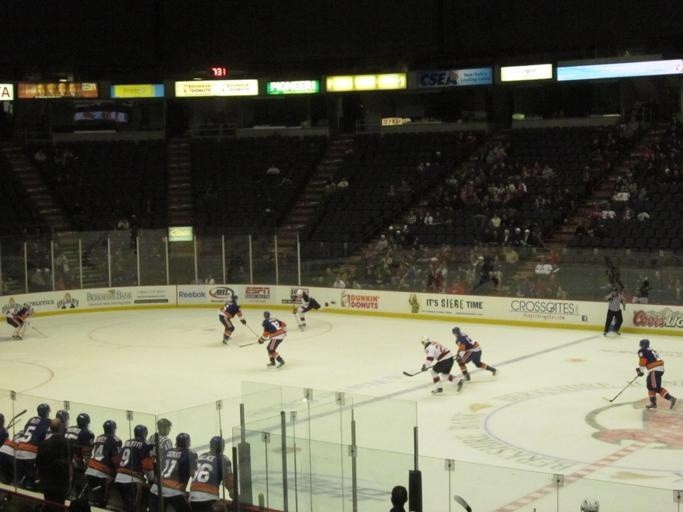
[603,375,638,402]
[403,371,423,376]
[235,340,257,346]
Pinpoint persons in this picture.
[452,327,497,381]
[7,307,32,340]
[390,485,408,512]
[295,290,319,329]
[219,294,246,345]
[636,338,678,410]
[258,311,287,368]
[421,336,464,394]
[602,287,626,335]
[0,404,234,512]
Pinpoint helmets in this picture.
[56,410,70,423]
[37,404,51,417]
[158,418,172,433]
[77,413,90,426]
[209,436,225,452]
[102,420,117,432]
[134,424,148,437]
[175,432,191,448]
